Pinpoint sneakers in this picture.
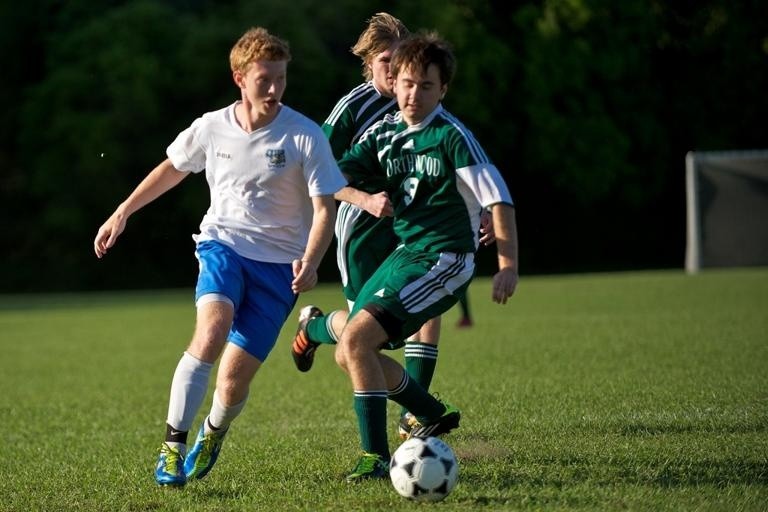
[291,304,324,372]
[182,416,230,479]
[153,442,187,486]
[346,450,391,483]
[407,400,461,443]
[398,410,419,439]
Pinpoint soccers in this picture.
[390,436,459,504]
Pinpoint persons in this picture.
[90,26,350,490]
[289,10,496,441]
[332,26,521,484]
